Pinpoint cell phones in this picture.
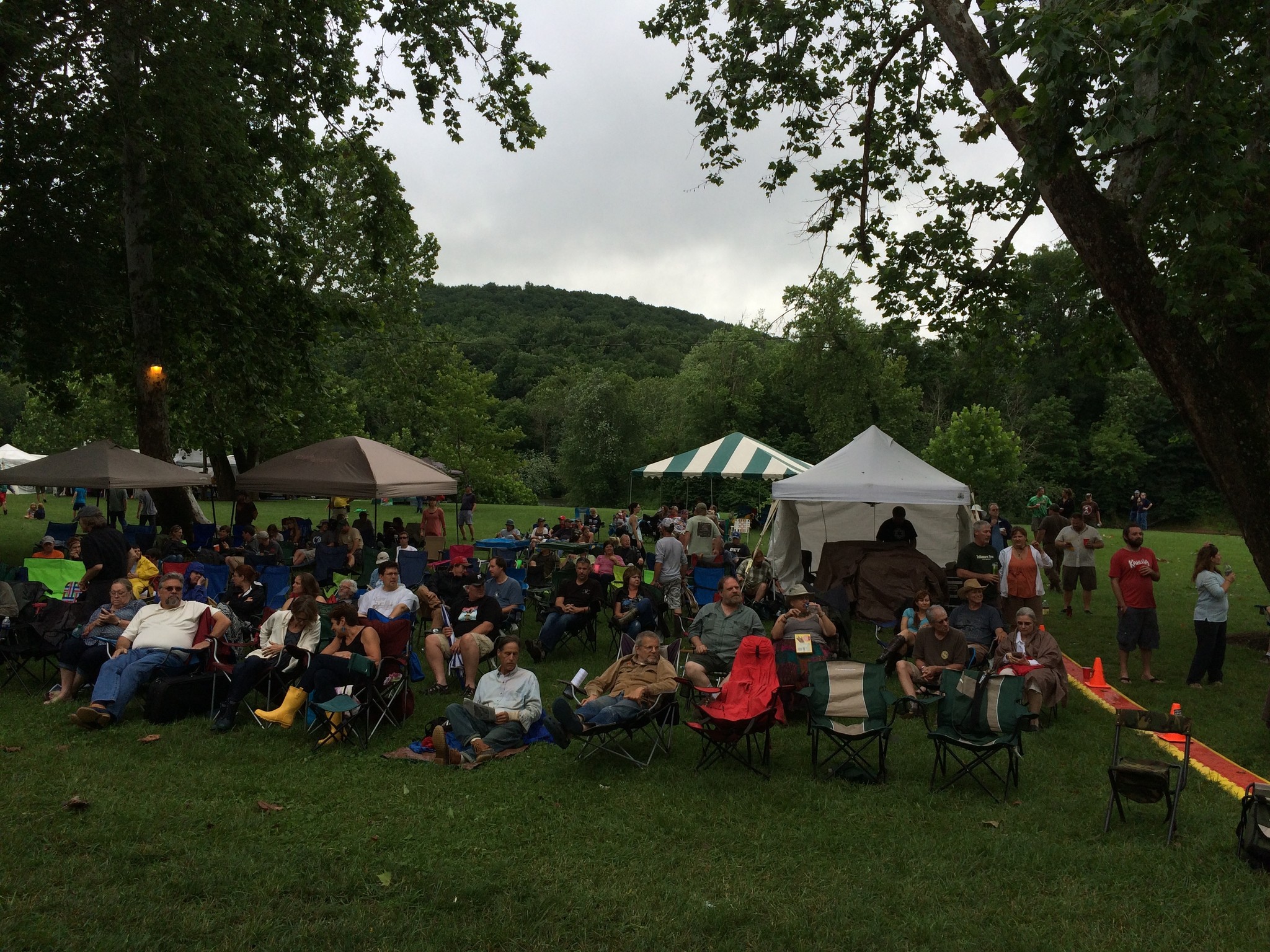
[1066,542,1073,548]
[1011,652,1023,659]
[101,609,111,615]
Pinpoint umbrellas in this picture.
[439,595,466,691]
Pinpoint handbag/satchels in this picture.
[615,523,639,542]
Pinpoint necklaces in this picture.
[1014,547,1024,557]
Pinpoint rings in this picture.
[105,618,106,620]
[455,648,457,649]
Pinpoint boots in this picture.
[209,698,239,732]
[876,634,906,676]
[254,685,309,730]
[612,607,639,632]
[318,711,347,745]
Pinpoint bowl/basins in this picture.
[999,527,1005,531]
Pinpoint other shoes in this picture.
[900,701,928,719]
[524,638,546,663]
[541,697,584,750]
[693,695,715,720]
[70,701,111,730]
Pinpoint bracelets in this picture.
[819,611,825,618]
[583,607,585,611]
[920,665,925,668]
[205,637,212,643]
[1225,579,1232,583]
[781,619,788,624]
[783,615,788,619]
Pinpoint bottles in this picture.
[805,604,819,614]
[992,562,998,575]
[1224,564,1232,577]
[197,546,201,552]
[1,616,10,640]
[1042,600,1049,615]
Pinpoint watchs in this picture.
[115,618,121,626]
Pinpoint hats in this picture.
[557,515,582,523]
[376,551,390,564]
[428,496,436,500]
[1134,490,1140,493]
[71,504,103,522]
[670,506,678,511]
[1085,493,1092,497]
[505,519,514,525]
[253,531,269,539]
[537,517,546,521]
[41,535,55,546]
[660,517,675,528]
[971,504,987,517]
[463,484,473,488]
[732,531,741,539]
[464,572,489,586]
[449,555,474,568]
[957,578,989,600]
[695,502,707,511]
[783,584,815,596]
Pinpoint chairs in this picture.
[924,668,1025,806]
[1103,708,1192,848]
[793,661,893,782]
[0,512,530,753]
[532,503,1001,670]
[571,633,682,768]
[685,634,779,781]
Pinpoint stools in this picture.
[1236,782,1270,862]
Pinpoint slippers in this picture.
[1119,677,1132,684]
[43,691,75,705]
[1148,676,1164,684]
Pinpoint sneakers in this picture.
[432,725,461,766]
[470,738,499,761]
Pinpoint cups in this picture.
[1036,503,1040,506]
[1083,538,1090,546]
[1097,523,1101,527]
[213,544,220,552]
[1135,563,1146,576]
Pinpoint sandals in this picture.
[463,684,476,700]
[424,680,450,696]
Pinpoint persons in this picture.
[1130,491,1153,530]
[1185,541,1236,689]
[0,486,1104,765]
[1108,523,1165,684]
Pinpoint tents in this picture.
[628,424,971,599]
[228,435,458,541]
[0,440,218,537]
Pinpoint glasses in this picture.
[638,646,661,652]
[339,585,351,590]
[160,586,183,591]
[399,536,408,539]
[384,572,399,576]
